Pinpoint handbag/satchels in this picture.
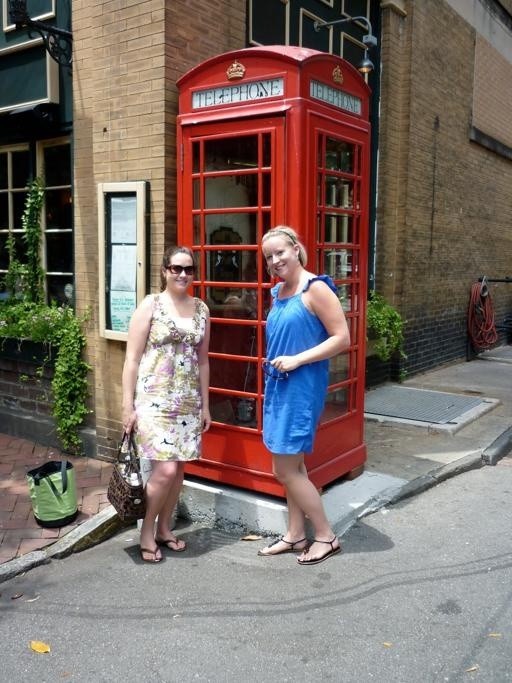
[108,454,145,523]
[26,460,78,527]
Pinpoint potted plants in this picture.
[366,288,407,382]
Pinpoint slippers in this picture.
[139,542,163,562]
[156,535,187,551]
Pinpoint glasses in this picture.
[166,265,195,275]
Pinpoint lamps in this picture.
[312,16,381,76]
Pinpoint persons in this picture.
[121,244,213,564]
[253,224,352,566]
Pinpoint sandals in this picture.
[258,536,307,556]
[297,535,341,564]
